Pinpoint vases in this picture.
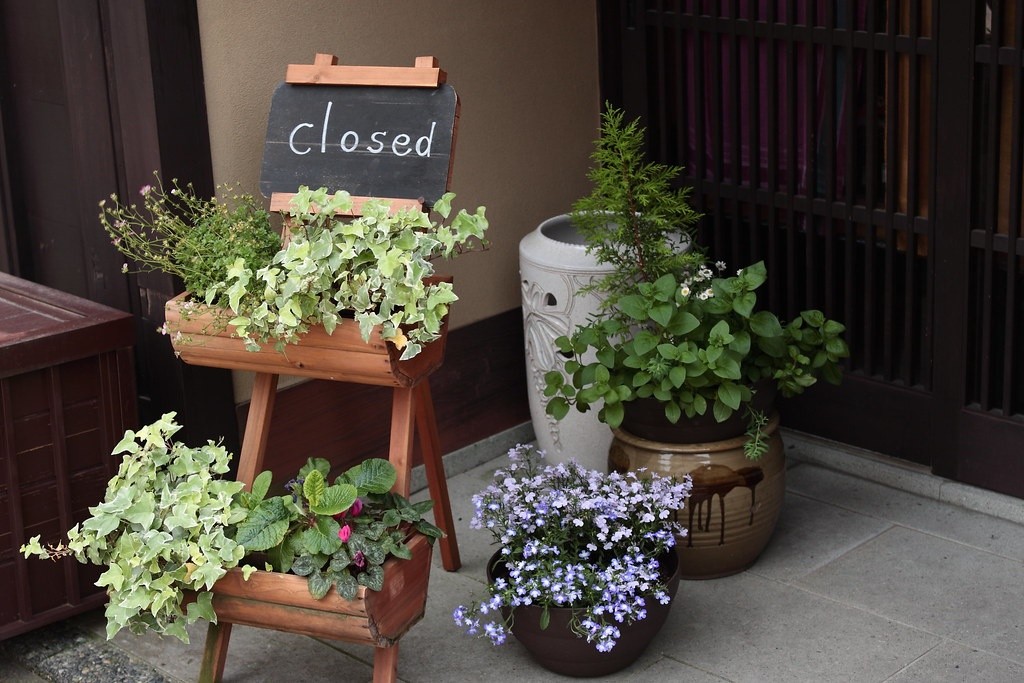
[178,517,438,647]
[488,538,681,677]
[519,209,690,474]
[166,274,456,387]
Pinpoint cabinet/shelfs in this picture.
[0,270,142,641]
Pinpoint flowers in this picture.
[454,443,692,655]
[100,168,493,361]
[18,411,449,644]
[544,254,853,461]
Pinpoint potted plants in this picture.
[568,101,786,580]
